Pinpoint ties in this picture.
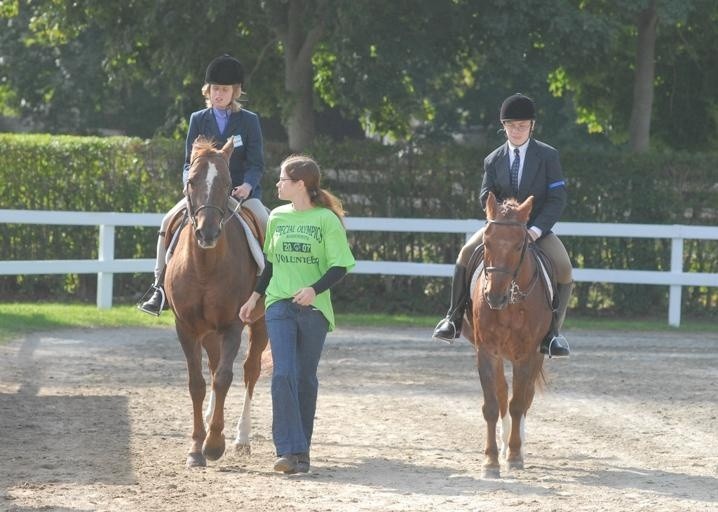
[511,149,519,192]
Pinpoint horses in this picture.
[459,191,557,480]
[164,134,271,468]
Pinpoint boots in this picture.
[433,265,466,338]
[540,279,572,355]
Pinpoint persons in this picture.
[434,93,574,357]
[140,53,266,315]
[237,155,356,475]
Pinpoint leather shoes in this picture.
[142,292,169,311]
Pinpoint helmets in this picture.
[205,54,244,85]
[500,93,535,121]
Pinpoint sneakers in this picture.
[274,452,309,474]
